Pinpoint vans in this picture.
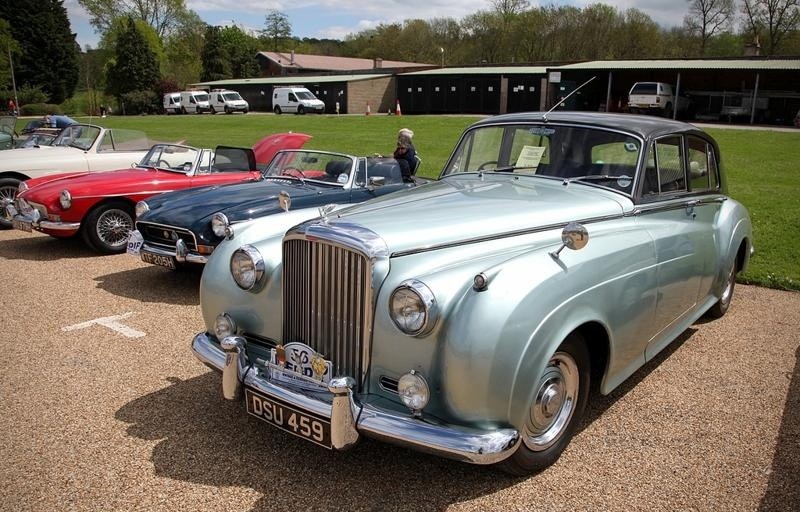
[208,88,250,114]
[162,92,182,116]
[271,87,326,115]
[180,90,209,115]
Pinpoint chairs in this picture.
[315,158,347,185]
[369,161,404,187]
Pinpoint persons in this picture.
[107,106,112,119]
[375,128,417,182]
[100,104,105,117]
[9,100,17,116]
[44,113,77,129]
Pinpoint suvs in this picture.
[626,81,695,117]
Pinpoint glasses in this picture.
[398,147,408,149]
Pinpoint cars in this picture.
[0,114,93,149]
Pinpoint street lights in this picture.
[439,46,445,68]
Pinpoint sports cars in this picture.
[131,145,422,278]
[0,123,234,231]
[191,77,755,482]
[11,134,330,257]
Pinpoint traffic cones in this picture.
[394,99,402,116]
[365,100,372,115]
